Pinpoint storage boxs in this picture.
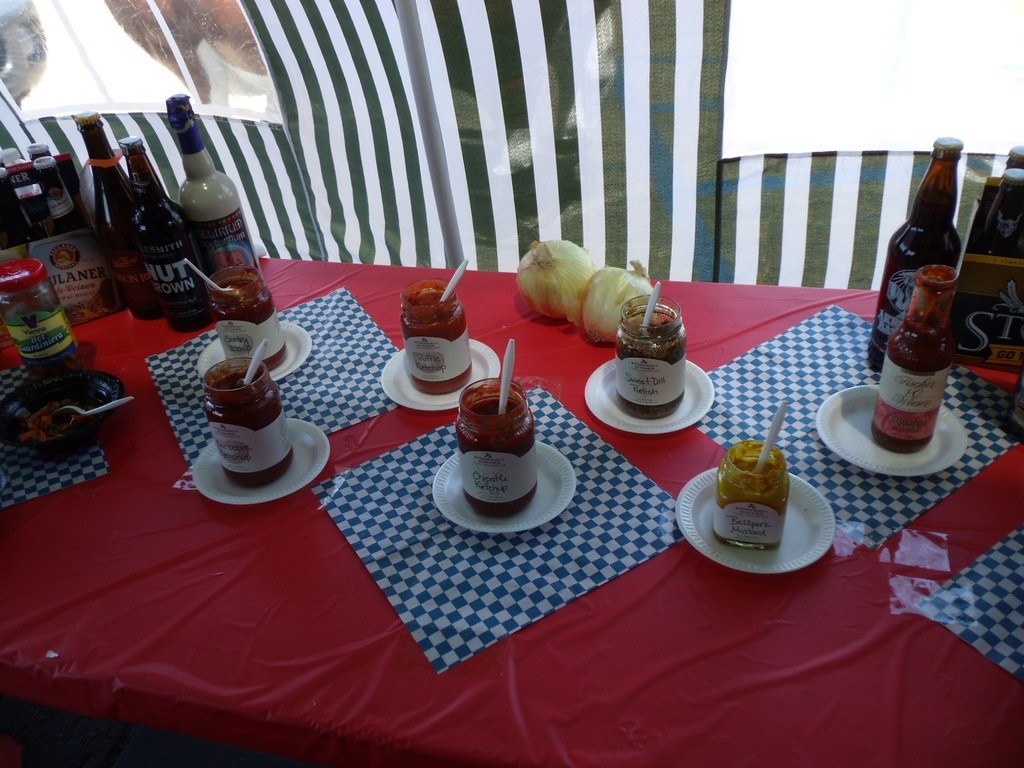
[0,154,123,353]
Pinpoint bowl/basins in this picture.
[0,371,126,460]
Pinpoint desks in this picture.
[0,258,1024,768]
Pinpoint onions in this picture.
[517,239,655,343]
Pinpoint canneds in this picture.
[712,441,790,551]
[456,377,538,516]
[207,265,288,371]
[615,294,688,419]
[1,259,80,375]
[203,357,293,487]
[401,278,473,394]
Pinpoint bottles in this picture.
[872,265,956,453]
[118,137,211,331]
[869,137,961,376]
[206,264,289,369]
[202,359,293,488]
[616,296,686,419]
[403,279,472,393]
[72,112,161,320]
[713,441,790,549]
[0,147,46,245]
[166,94,260,277]
[33,157,86,235]
[456,379,536,518]
[983,147,1024,254]
[27,142,75,193]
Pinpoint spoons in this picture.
[53,395,134,417]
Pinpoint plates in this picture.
[677,467,835,573]
[197,322,314,383]
[381,337,501,412]
[432,439,576,531]
[585,358,715,434]
[817,385,968,478]
[192,420,331,506]
